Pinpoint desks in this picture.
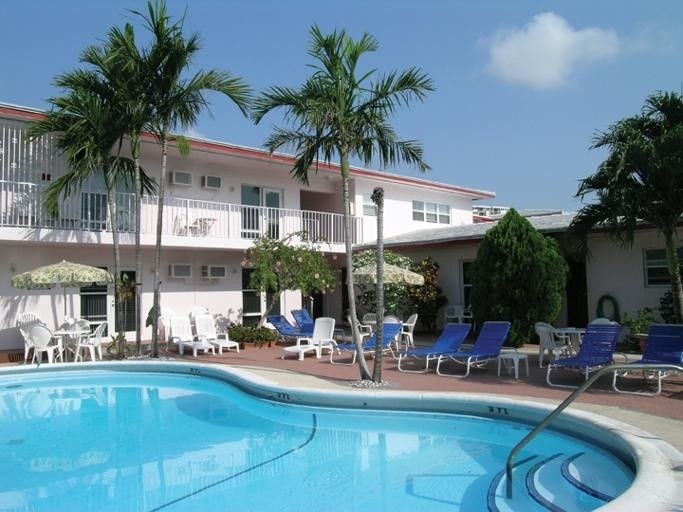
[195,217,218,236]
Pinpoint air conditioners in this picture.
[168,263,193,279]
[169,170,193,187]
[201,175,222,189]
[201,265,227,279]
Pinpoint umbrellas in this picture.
[11,259,116,315]
[345,261,424,287]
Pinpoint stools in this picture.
[497,352,529,380]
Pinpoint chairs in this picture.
[546,325,627,389]
[533,319,611,369]
[195,307,239,354]
[437,322,517,378]
[397,323,472,374]
[457,304,477,330]
[267,308,418,361]
[17,313,108,365]
[612,324,683,396]
[176,211,199,237]
[443,305,464,327]
[170,308,215,357]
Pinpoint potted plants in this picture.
[228,321,279,350]
[623,308,655,351]
